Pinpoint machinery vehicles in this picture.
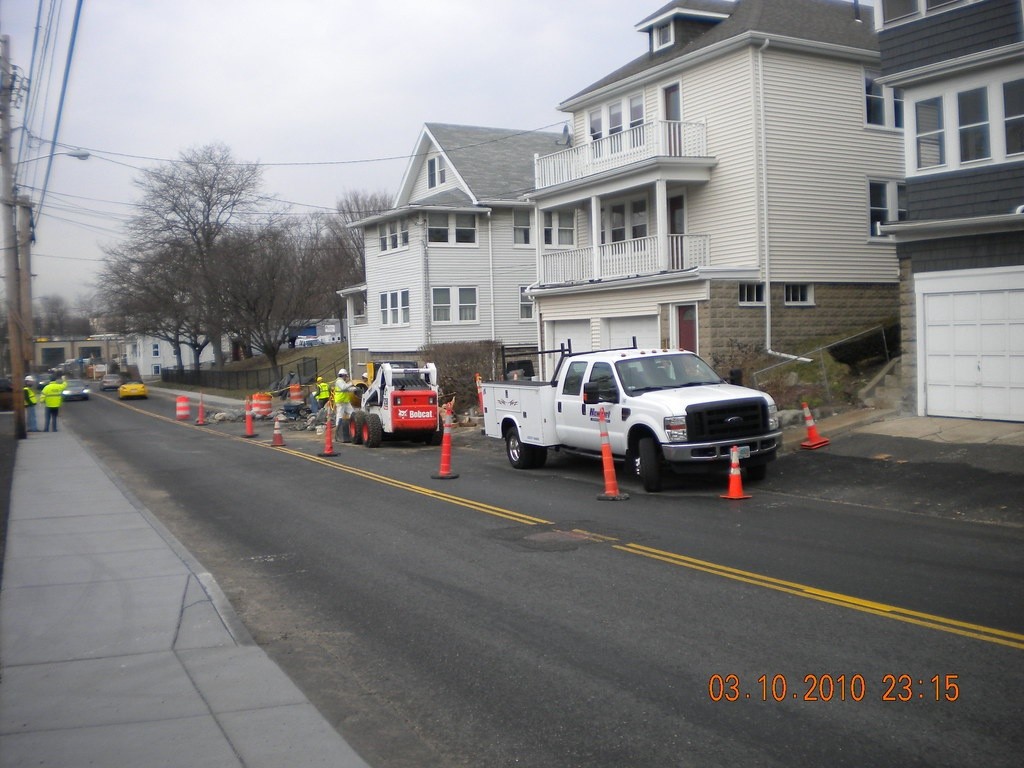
[338,359,444,447]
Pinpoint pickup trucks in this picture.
[480,334,783,493]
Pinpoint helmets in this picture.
[316,376,323,382]
[24,375,34,381]
[338,368,347,375]
[362,372,368,378]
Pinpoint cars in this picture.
[61,380,90,401]
[5,375,13,390]
[98,374,122,391]
[48,359,109,378]
[37,373,55,388]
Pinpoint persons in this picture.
[40,375,67,432]
[334,368,359,429]
[362,373,369,384]
[23,376,41,433]
[312,376,332,408]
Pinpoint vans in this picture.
[296,335,342,347]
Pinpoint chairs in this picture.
[648,368,673,387]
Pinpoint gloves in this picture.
[349,382,353,386]
[350,386,357,391]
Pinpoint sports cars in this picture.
[117,381,148,399]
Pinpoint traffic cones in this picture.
[721,444,753,499]
[799,403,828,450]
[271,418,286,448]
